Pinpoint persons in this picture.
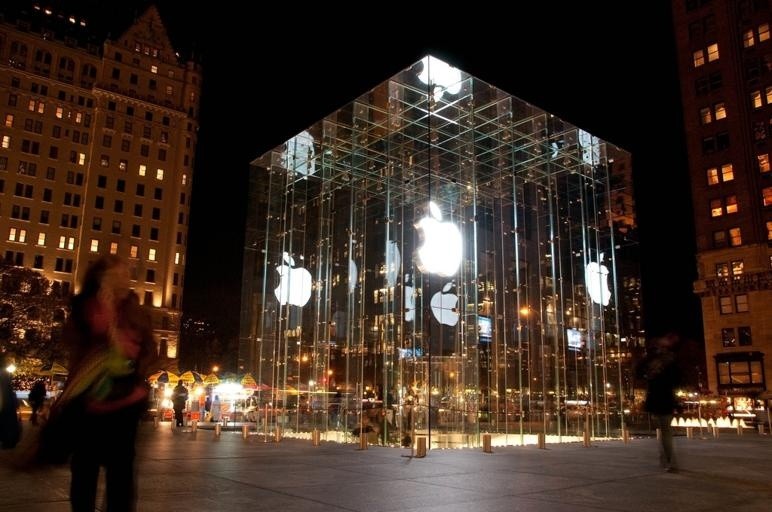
[35,257,161,511]
[205,397,211,412]
[644,360,681,473]
[211,395,221,422]
[233,396,257,422]
[172,380,188,427]
[1,352,66,448]
